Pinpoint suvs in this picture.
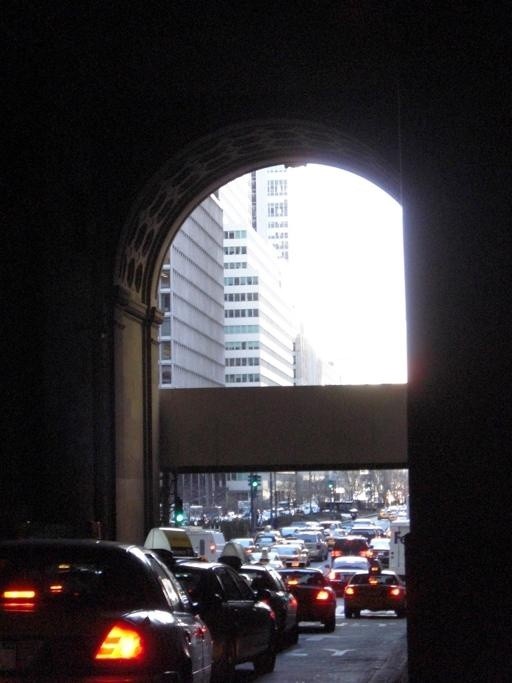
[320,521,346,535]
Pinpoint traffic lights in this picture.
[327,480,335,493]
[174,498,184,527]
[248,475,261,489]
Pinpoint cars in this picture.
[237,565,298,647]
[0,538,209,683]
[327,557,373,597]
[348,506,406,562]
[228,520,319,551]
[271,543,309,568]
[169,562,279,679]
[276,568,336,631]
[343,572,408,618]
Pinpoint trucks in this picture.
[144,525,224,567]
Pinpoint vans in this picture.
[325,536,372,556]
[295,532,328,561]
